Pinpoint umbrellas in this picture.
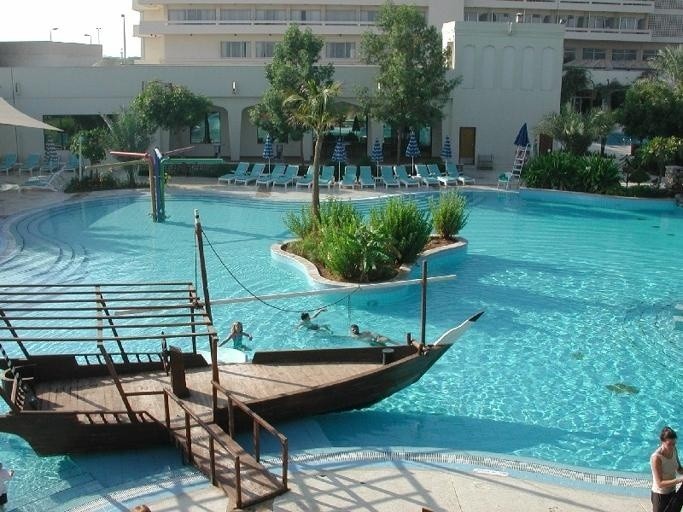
[370,136,384,180]
[43,136,58,172]
[438,134,452,174]
[404,130,423,176]
[513,121,532,148]
[261,132,275,177]
[331,138,350,182]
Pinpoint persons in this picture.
[294,307,333,340]
[648,425,683,512]
[218,318,253,349]
[0,463,15,509]
[132,504,152,512]
[350,323,400,349]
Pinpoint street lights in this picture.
[49,13,126,59]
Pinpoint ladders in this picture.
[510,143,529,185]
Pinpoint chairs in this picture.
[497,172,512,190]
[216,159,476,192]
[0,152,84,192]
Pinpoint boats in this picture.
[0,209,484,510]
